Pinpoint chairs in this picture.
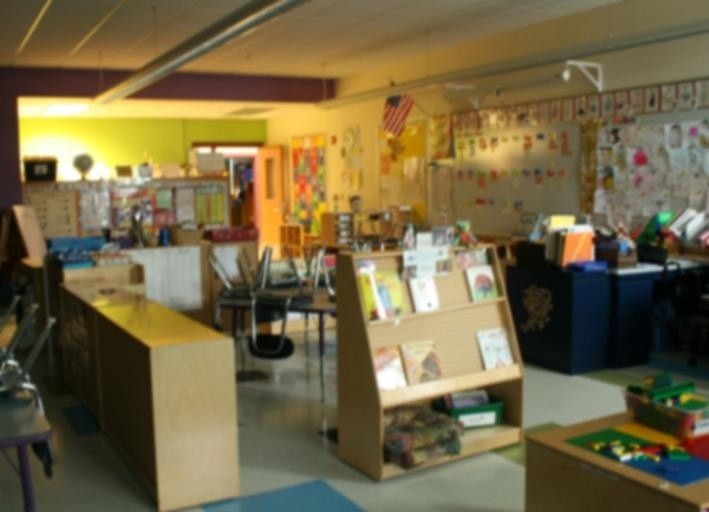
[257,245,385,403]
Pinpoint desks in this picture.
[509,255,701,374]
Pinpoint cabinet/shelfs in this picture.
[524,393,709,512]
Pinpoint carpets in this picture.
[177,480,384,511]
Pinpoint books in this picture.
[361,269,404,322]
[407,275,440,313]
[534,208,708,270]
[371,345,406,393]
[477,328,515,370]
[399,336,441,387]
[403,222,476,279]
[465,263,500,302]
